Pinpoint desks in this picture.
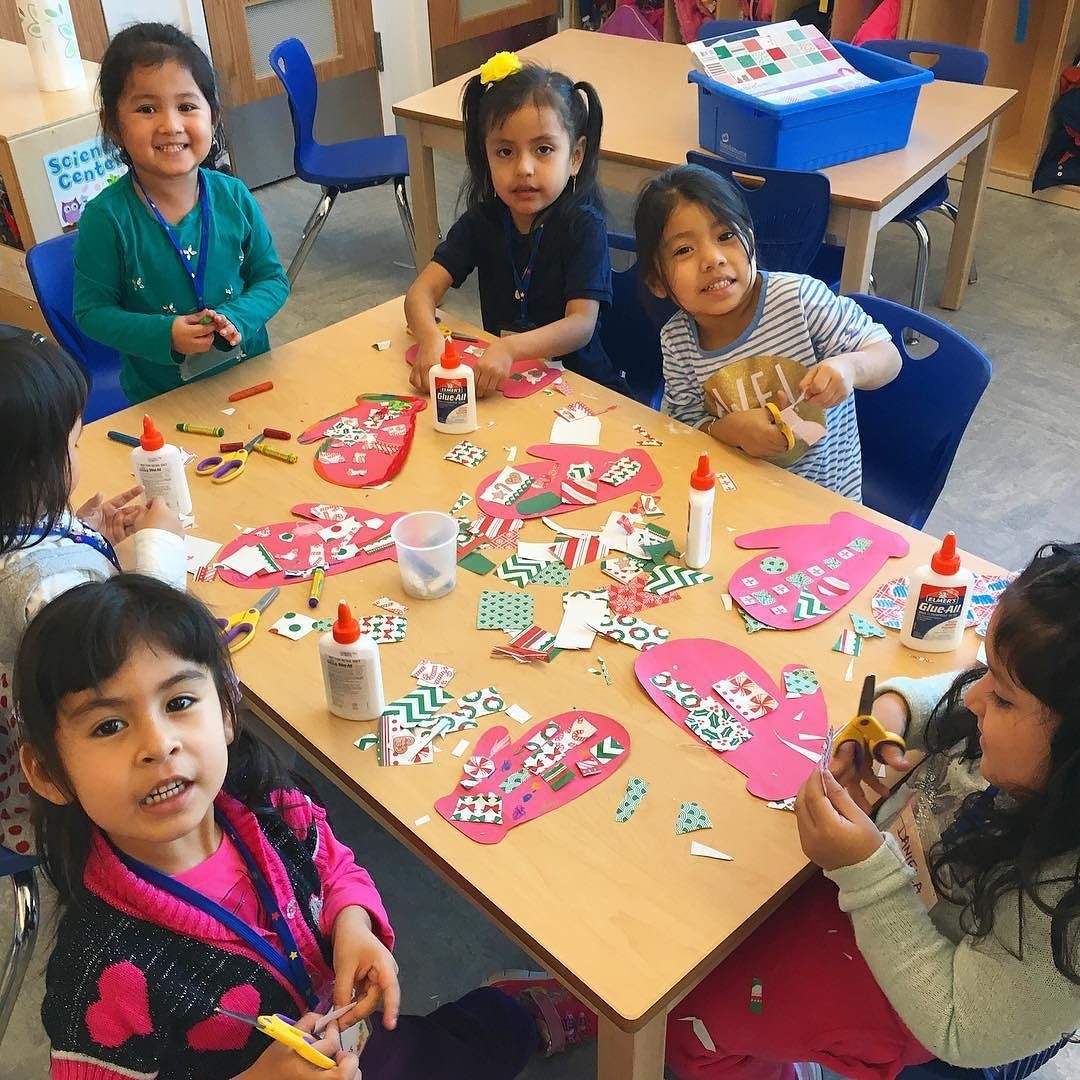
[50,287,1041,1080]
[390,28,1021,353]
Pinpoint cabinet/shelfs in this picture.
[551,1,1080,212]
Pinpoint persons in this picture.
[630,163,903,511]
[664,540,1079,1080]
[0,325,327,874]
[6,568,598,1079]
[70,22,292,406]
[399,49,627,398]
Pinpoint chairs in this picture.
[537,228,701,411]
[786,291,995,536]
[681,150,879,317]
[26,230,151,427]
[265,34,447,296]
[825,37,990,350]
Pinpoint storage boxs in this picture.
[682,41,936,178]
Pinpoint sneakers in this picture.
[480,968,599,1059]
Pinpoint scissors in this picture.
[212,586,281,657]
[406,316,478,342]
[829,673,907,772]
[194,433,264,484]
[765,402,795,453]
[215,1007,338,1071]
